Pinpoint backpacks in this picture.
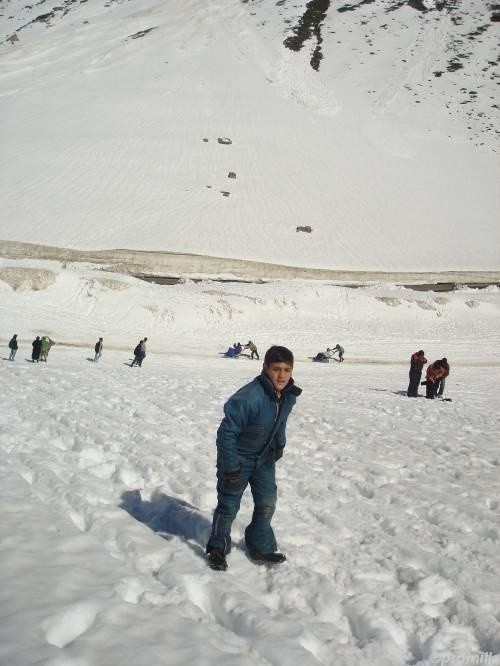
[8,339,14,350]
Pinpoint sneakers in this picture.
[408,394,443,398]
[32,359,47,363]
[252,551,287,564]
[339,358,344,362]
[131,363,141,367]
[206,547,229,570]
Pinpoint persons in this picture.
[40,336,56,362]
[32,336,42,363]
[333,344,345,362]
[407,350,427,397]
[315,348,331,362]
[205,346,301,572]
[9,335,18,360]
[426,362,446,398]
[94,338,103,361]
[434,358,450,397]
[245,341,259,360]
[130,340,144,367]
[226,343,241,354]
[135,337,148,364]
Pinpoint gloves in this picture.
[275,444,285,463]
[223,470,241,491]
